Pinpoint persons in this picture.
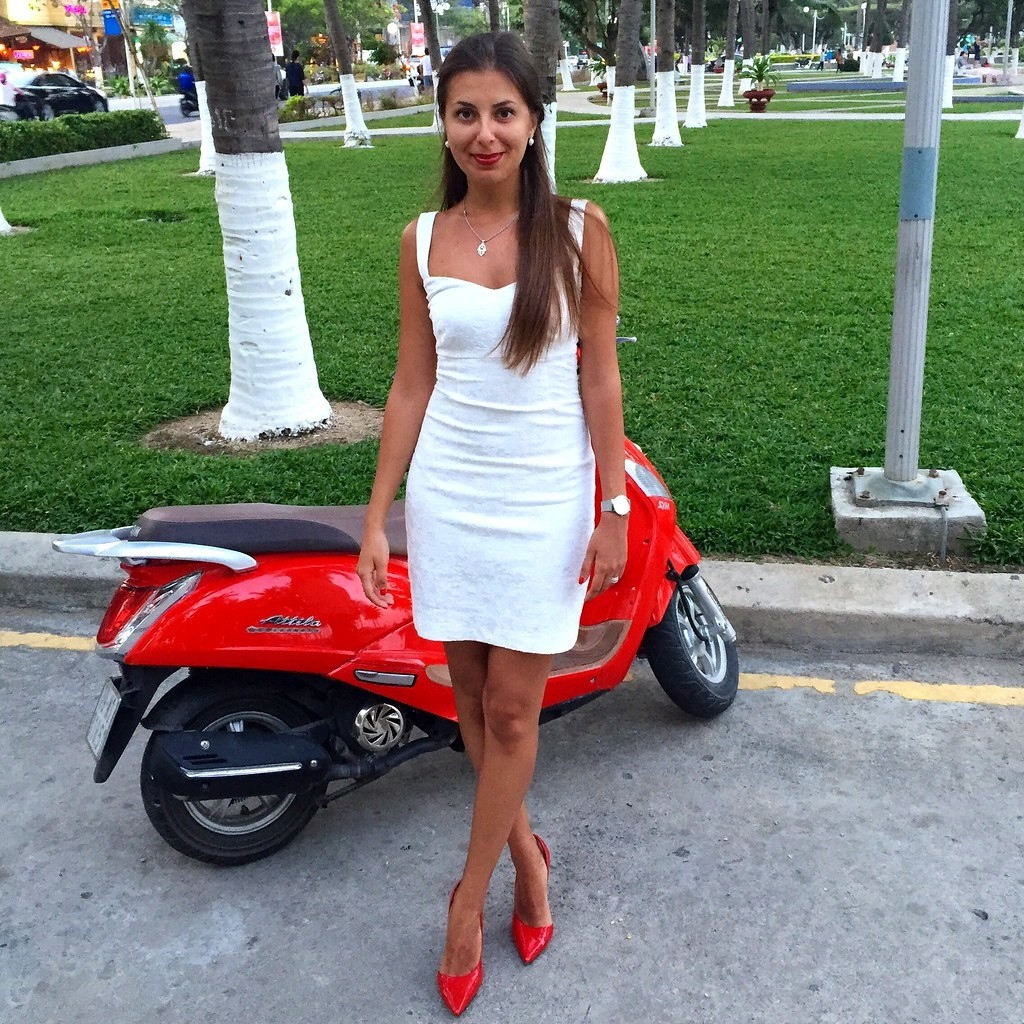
[835,49,844,73]
[817,49,825,70]
[420,47,433,89]
[179,66,196,95]
[271,53,283,100]
[0,74,23,107]
[827,48,833,71]
[286,51,309,98]
[975,42,982,66]
[355,31,630,1018]
[958,50,968,75]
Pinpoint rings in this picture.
[611,576,619,584]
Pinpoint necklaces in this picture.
[462,194,519,257]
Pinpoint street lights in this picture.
[803,5,827,59]
[431,0,450,46]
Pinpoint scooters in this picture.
[0,93,40,125]
[178,90,200,119]
[51,315,741,866]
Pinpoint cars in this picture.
[1,61,29,92]
[577,54,602,70]
[272,67,289,102]
[566,55,579,67]
[705,53,744,73]
[12,72,108,122]
[404,48,451,88]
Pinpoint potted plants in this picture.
[584,56,607,97]
[734,55,783,114]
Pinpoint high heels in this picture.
[510,834,554,964]
[438,879,485,1017]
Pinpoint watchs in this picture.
[600,495,631,517]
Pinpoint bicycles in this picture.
[328,75,362,110]
